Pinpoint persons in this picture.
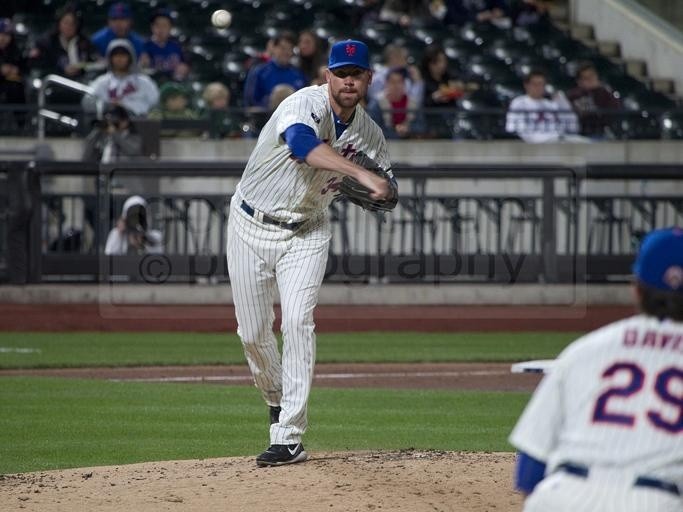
[507,228,683,511]
[0,0,631,276]
[228,40,397,465]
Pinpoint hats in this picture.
[328,40,370,70]
[633,226,682,292]
[107,3,130,18]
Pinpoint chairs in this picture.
[0,0,683,172]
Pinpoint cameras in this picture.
[92,118,120,130]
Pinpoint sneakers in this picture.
[257,443,307,465]
[270,406,281,423]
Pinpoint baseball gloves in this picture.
[337,150,399,214]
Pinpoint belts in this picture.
[559,462,678,494]
[240,202,308,230]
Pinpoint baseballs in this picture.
[211,10,232,29]
